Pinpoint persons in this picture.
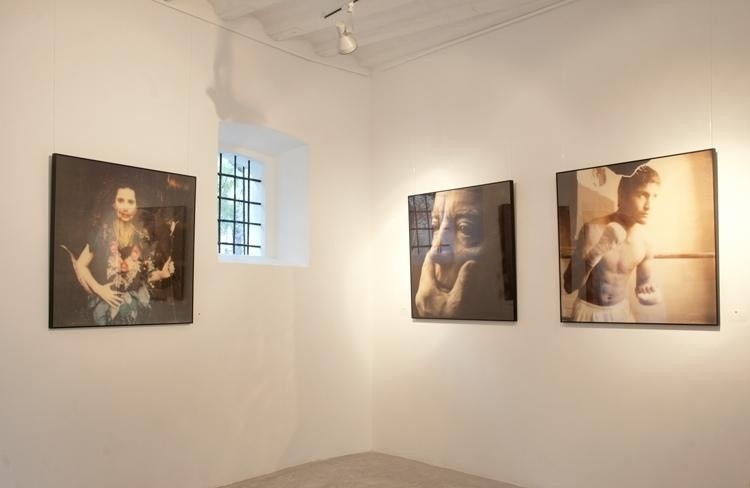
[74,183,175,325]
[563,166,662,325]
[413,185,501,319]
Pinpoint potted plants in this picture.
[50,153,197,329]
[406,178,517,321]
[555,148,719,327]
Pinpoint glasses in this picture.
[325,0,362,54]
